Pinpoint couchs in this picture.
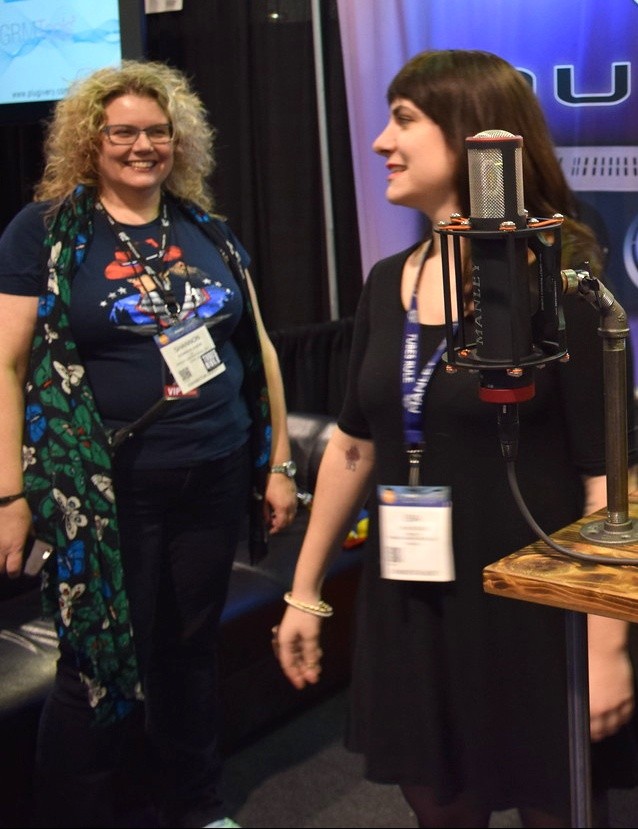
[0,414,369,829]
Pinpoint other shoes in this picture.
[158,804,242,829]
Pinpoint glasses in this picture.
[101,123,173,145]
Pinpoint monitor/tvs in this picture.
[0,0,145,122]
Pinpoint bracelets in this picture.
[283,591,332,617]
[0,492,24,505]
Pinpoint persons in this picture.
[0,59,297,829]
[276,53,638,829]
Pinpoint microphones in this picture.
[466,129,537,463]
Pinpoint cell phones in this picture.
[23,536,54,577]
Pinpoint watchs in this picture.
[268,461,296,478]
